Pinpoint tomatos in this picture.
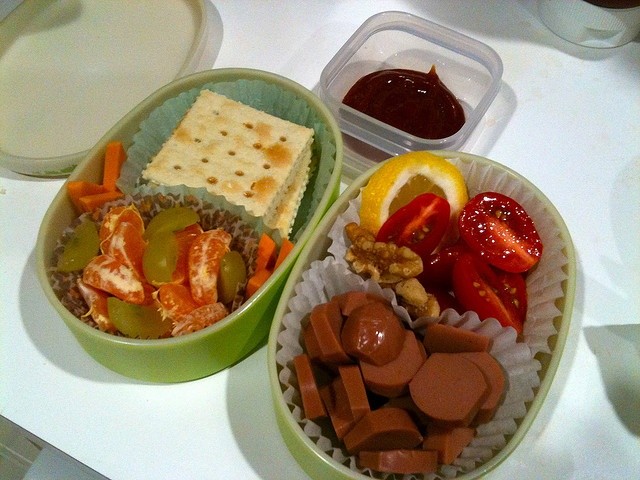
[452,256,526,337]
[422,247,464,277]
[373,193,450,259]
[459,193,544,273]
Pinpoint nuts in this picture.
[397,279,440,320]
[343,222,422,286]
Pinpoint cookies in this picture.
[142,89,314,222]
[267,151,312,238]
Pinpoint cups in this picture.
[538,1,638,50]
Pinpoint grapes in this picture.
[146,208,200,230]
[105,294,172,340]
[55,221,99,274]
[219,252,248,303]
[142,230,178,285]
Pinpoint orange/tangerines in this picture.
[155,285,196,325]
[82,256,145,306]
[188,229,232,305]
[109,222,148,283]
[167,226,203,285]
[170,302,226,335]
[99,205,126,255]
[75,278,116,331]
